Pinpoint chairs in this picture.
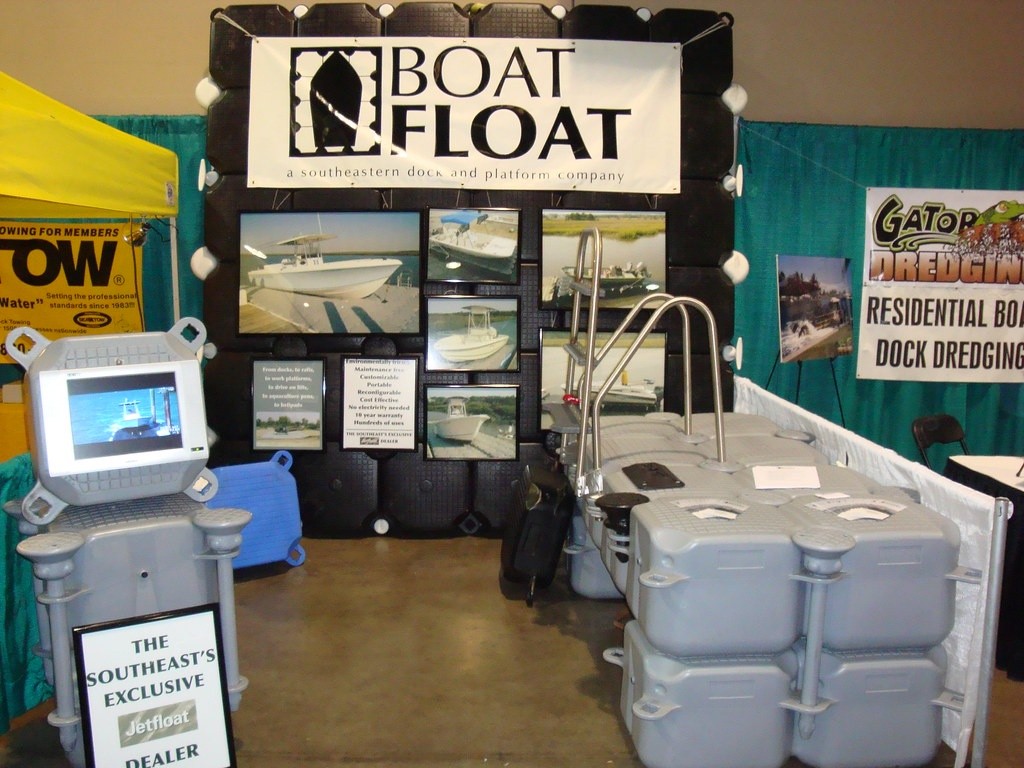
[911,413,970,473]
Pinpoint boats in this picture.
[429,222,519,262]
[247,233,401,301]
[563,263,646,299]
[436,305,511,363]
[117,397,151,429]
[561,379,657,408]
[428,399,491,444]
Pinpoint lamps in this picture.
[121,215,152,248]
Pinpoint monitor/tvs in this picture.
[67,372,184,461]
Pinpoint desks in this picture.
[942,455,1024,683]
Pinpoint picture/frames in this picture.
[425,205,522,286]
[538,327,668,432]
[339,354,419,453]
[235,210,424,338]
[423,383,520,462]
[425,294,521,372]
[248,356,328,454]
[539,207,671,312]
